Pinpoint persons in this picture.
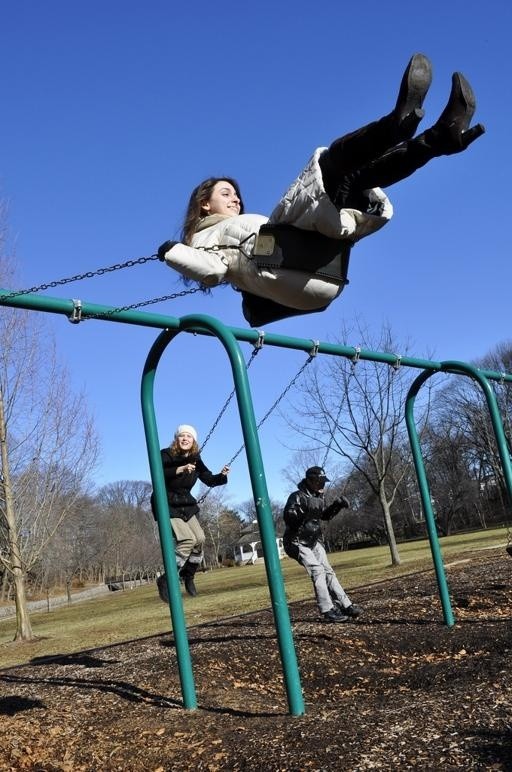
[147,423,232,603]
[286,464,362,623]
[174,53,488,328]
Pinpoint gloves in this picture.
[156,237,180,263]
[335,495,351,509]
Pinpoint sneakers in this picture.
[323,610,348,623]
[340,604,362,616]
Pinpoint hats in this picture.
[305,466,332,483]
[173,424,199,444]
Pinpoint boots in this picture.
[155,563,182,605]
[319,52,432,207]
[334,69,486,213]
[179,551,202,597]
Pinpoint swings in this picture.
[151,345,315,521]
[283,361,398,564]
[1,225,353,320]
[470,377,512,555]
